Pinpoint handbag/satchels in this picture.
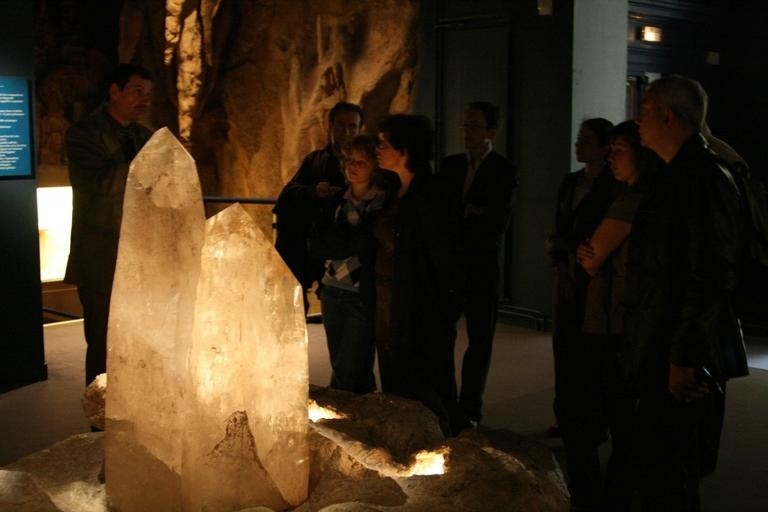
[276,210,326,287]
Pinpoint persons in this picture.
[539,72,768,511]
[61,64,156,389]
[272,101,515,433]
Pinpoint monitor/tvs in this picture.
[0,73,38,180]
